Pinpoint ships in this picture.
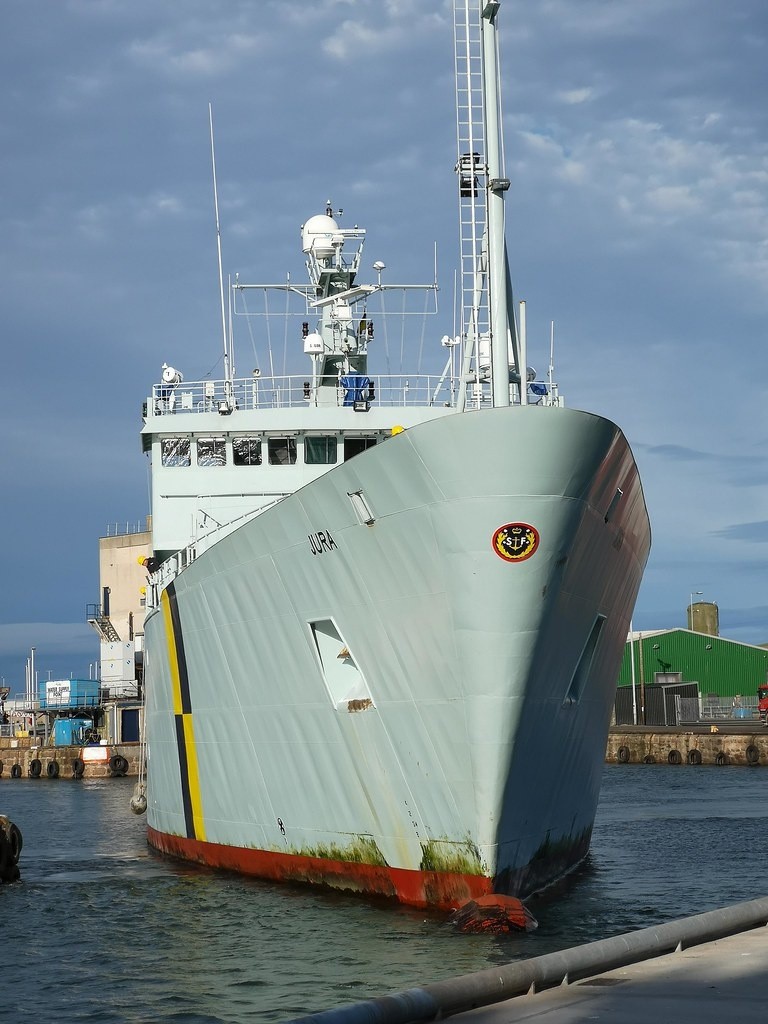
[137,1,656,926]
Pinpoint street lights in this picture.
[25,647,36,709]
[689,591,703,632]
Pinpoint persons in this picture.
[138,555,159,574]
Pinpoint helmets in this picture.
[392,425,403,437]
